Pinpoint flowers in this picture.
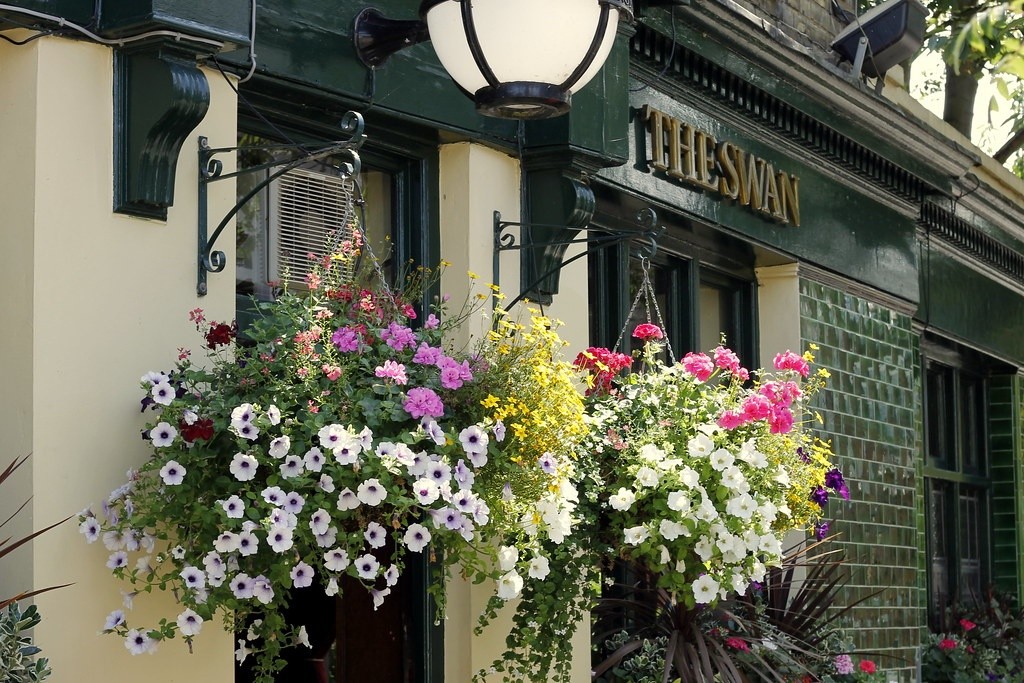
[74,219,1024,680]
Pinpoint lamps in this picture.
[345,0,635,121]
